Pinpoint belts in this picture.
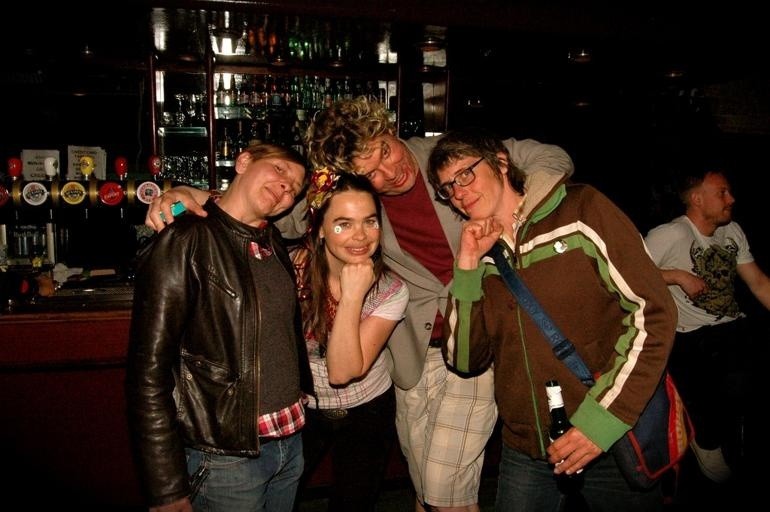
[314,408,347,421]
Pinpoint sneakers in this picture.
[690,438,730,481]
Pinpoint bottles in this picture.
[174,7,211,56]
[211,1,378,192]
[544,378,586,497]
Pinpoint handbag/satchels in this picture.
[594,365,698,492]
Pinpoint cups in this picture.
[158,92,210,190]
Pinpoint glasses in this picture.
[437,156,487,201]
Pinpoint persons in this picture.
[642,163,769,485]
[279,169,414,512]
[143,90,578,512]
[422,128,680,512]
[124,136,314,512]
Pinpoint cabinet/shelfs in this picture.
[149,2,401,194]
